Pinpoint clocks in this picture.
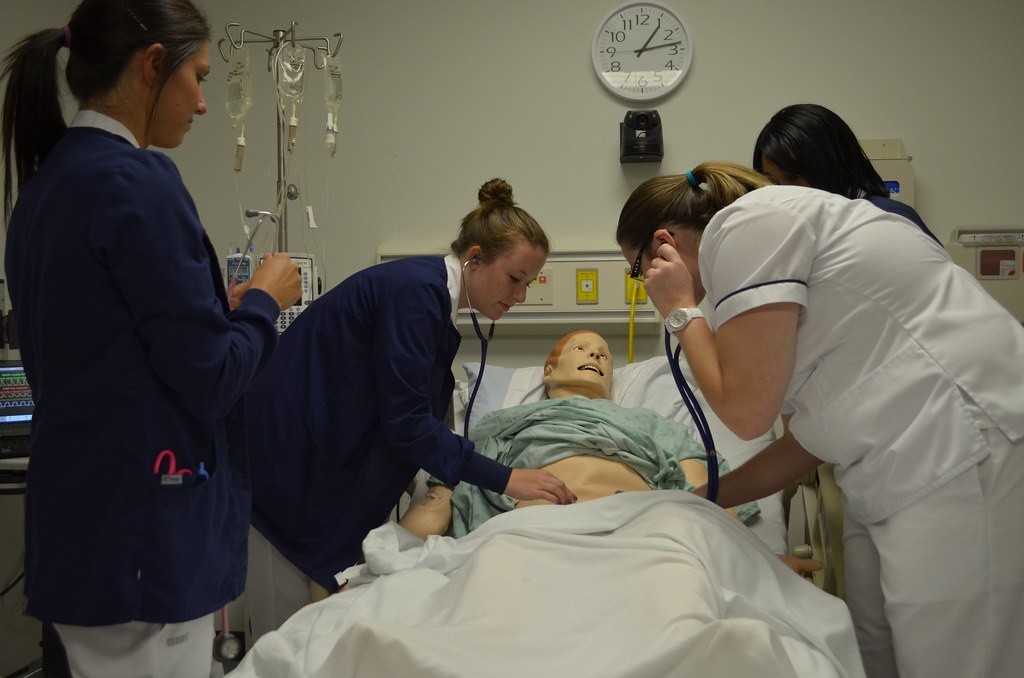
[591,1,693,102]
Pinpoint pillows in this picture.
[463,356,702,445]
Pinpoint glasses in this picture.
[630,232,675,282]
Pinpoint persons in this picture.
[752,104,942,245]
[399,330,823,577]
[1,1,305,678]
[248,176,578,637]
[617,162,1024,661]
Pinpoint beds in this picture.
[218,373,866,677]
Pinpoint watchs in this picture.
[664,307,706,334]
